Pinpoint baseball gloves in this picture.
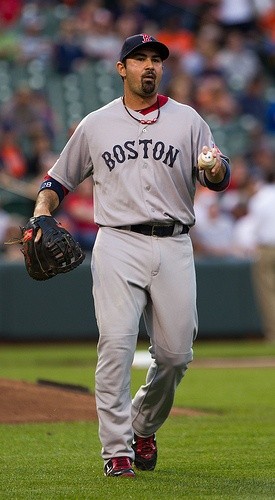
[18,215,86,281]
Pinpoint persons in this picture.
[0,0,275,344]
[33,33,231,477]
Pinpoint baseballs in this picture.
[197,150,218,171]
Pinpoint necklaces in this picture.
[123,95,160,125]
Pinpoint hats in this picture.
[119,33,170,63]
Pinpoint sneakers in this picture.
[131,432,158,471]
[104,456,135,477]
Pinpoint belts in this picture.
[97,223,191,237]
